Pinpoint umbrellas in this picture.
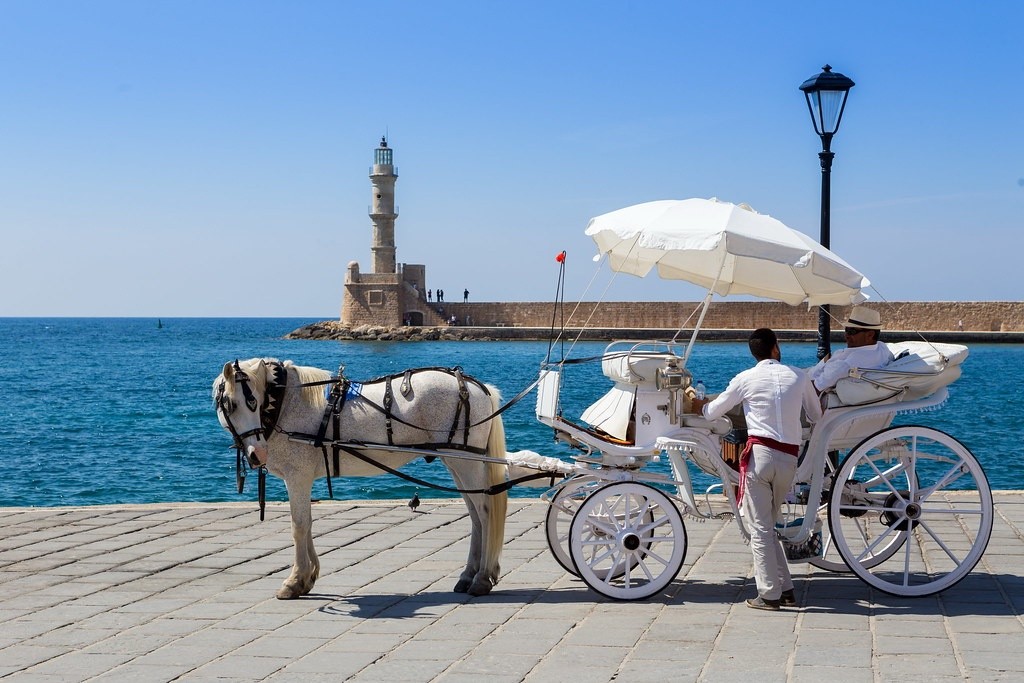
[584,196,871,366]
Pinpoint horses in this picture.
[211,356,508,600]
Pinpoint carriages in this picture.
[209,195,996,605]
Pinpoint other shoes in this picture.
[779,593,796,606]
[745,596,780,610]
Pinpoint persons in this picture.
[413,283,417,288]
[451,313,456,325]
[437,306,443,315]
[428,289,432,302]
[464,289,469,303]
[405,313,412,326]
[691,305,895,611]
[437,289,443,302]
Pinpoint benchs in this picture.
[579,340,682,445]
[796,338,969,456]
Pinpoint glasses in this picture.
[845,328,866,335]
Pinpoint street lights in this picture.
[796,63,857,504]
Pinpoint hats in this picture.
[841,306,884,330]
